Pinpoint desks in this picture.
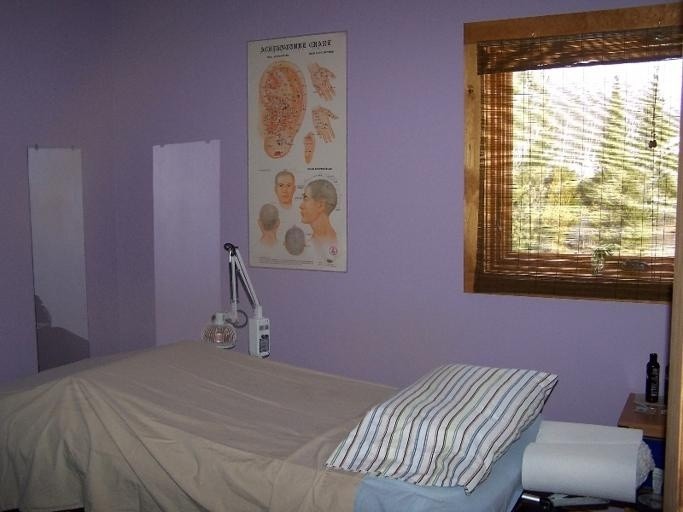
[616,390,666,466]
[508,432,663,510]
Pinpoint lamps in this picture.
[198,242,273,359]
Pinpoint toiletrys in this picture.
[653,467,663,494]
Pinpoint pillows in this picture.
[319,360,558,495]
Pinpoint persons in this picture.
[299,181,338,258]
[308,63,336,102]
[312,107,338,142]
[284,229,306,255]
[257,204,282,243]
[274,171,296,209]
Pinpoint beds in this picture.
[0,334,559,512]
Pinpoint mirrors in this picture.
[24,140,93,374]
